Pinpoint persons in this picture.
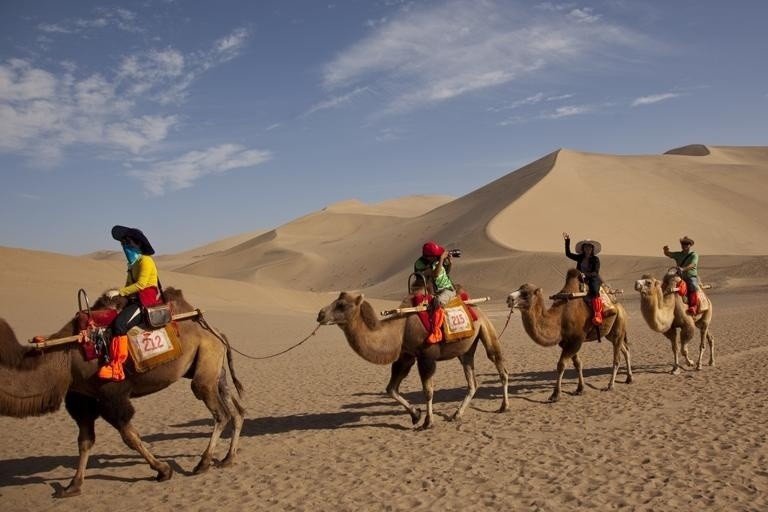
[662,236,697,314]
[96,224,158,381]
[562,231,601,326]
[412,240,452,345]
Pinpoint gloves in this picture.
[106,290,119,298]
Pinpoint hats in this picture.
[680,236,694,246]
[575,240,601,255]
[423,241,445,257]
[111,225,155,255]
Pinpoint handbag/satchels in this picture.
[145,304,172,329]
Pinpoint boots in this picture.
[592,298,602,325]
[689,292,697,316]
[97,336,128,381]
[427,308,444,344]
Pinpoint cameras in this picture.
[445,248,461,268]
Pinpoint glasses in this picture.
[121,239,131,246]
